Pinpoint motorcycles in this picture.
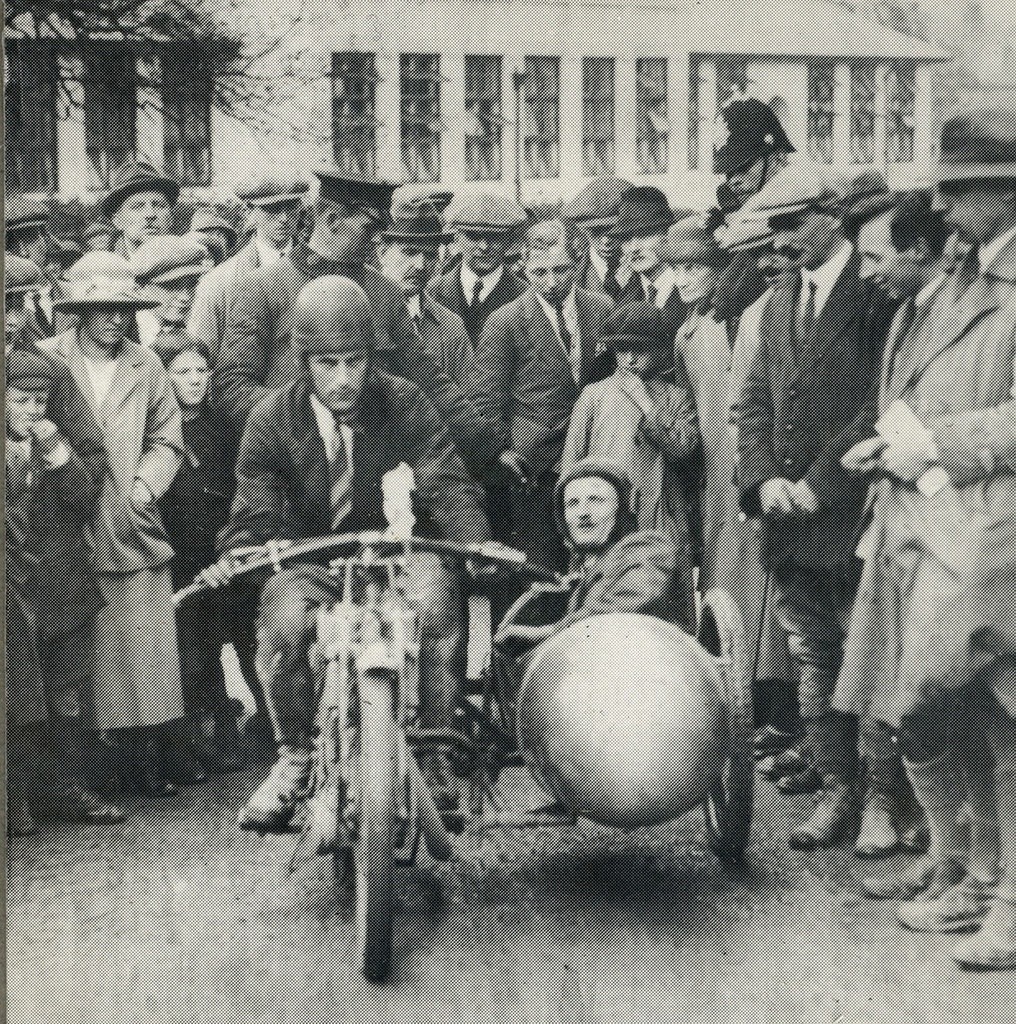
[172,526,759,979]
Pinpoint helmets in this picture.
[289,277,380,355]
[710,95,796,177]
[552,459,634,554]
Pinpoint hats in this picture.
[3,107,1016,394]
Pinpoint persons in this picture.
[5,98,1015,971]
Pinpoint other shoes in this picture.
[5,680,1016,976]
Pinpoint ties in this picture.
[886,299,916,386]
[798,280,817,344]
[325,414,354,535]
[950,244,982,309]
[555,304,571,355]
[472,281,484,307]
[603,259,622,298]
